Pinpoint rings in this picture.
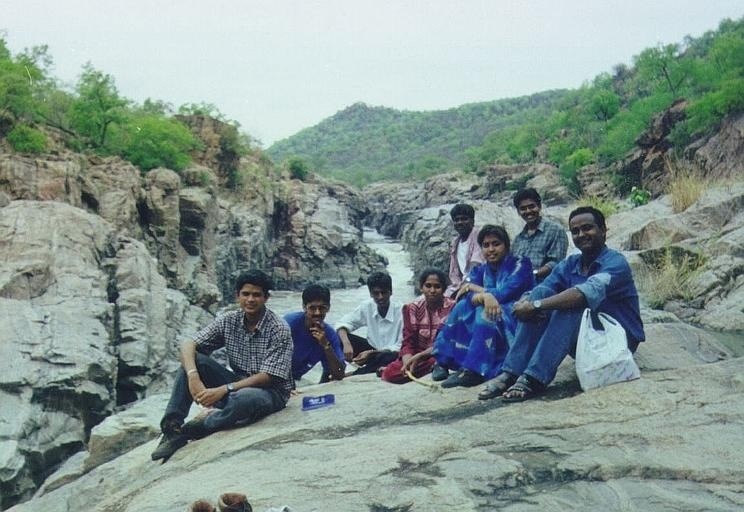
[315,331,319,336]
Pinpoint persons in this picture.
[381,188,567,387]
[283,285,346,398]
[152,268,294,460]
[318,271,403,384]
[477,205,644,402]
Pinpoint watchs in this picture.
[531,299,542,311]
[227,383,235,392]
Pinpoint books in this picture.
[301,392,336,410]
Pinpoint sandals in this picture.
[502,374,540,402]
[478,371,519,398]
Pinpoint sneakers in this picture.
[152,429,188,460]
[441,367,465,388]
[431,362,448,380]
[180,408,215,440]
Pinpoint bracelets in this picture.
[371,349,373,353]
[324,341,331,351]
[186,369,198,375]
